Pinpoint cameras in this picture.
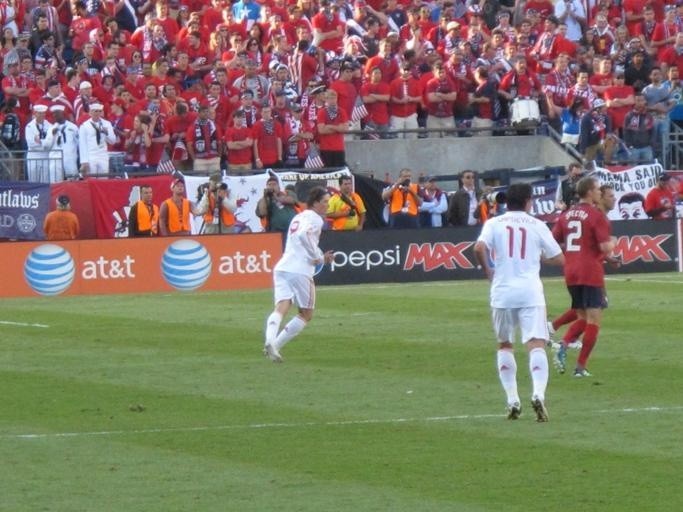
[349,210,354,216]
[483,191,505,204]
[264,188,274,197]
[211,182,227,192]
[400,179,411,186]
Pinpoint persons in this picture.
[262,187,329,364]
[473,186,565,421]
[551,178,613,379]
[1,0,682,241]
[546,185,616,351]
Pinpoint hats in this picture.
[89,102,104,109]
[50,104,66,112]
[267,176,278,183]
[269,59,279,71]
[33,104,48,112]
[170,179,184,190]
[447,21,460,30]
[425,174,438,182]
[659,173,671,181]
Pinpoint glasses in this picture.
[464,176,475,179]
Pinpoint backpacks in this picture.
[1,112,20,146]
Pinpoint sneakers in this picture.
[547,321,555,346]
[573,366,592,377]
[531,394,549,422]
[568,341,583,349]
[264,340,283,363]
[507,400,522,420]
[551,342,566,374]
